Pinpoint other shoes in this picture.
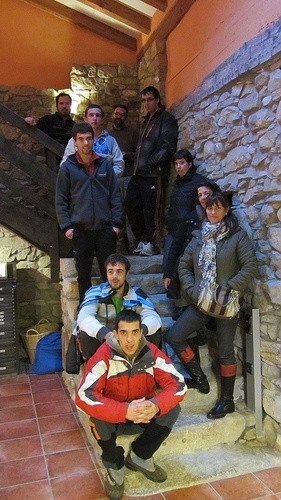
[125,447,166,484]
[103,462,130,500]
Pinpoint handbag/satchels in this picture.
[64,327,85,374]
[196,281,242,320]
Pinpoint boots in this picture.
[208,362,237,419]
[178,339,206,397]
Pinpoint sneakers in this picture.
[133,241,158,256]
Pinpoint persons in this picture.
[107,105,139,203]
[56,122,124,311]
[125,86,178,256]
[76,310,188,500]
[161,149,215,270]
[25,93,78,174]
[166,194,258,419]
[59,105,124,178]
[163,181,224,367]
[77,254,163,364]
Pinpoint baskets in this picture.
[23,318,61,363]
